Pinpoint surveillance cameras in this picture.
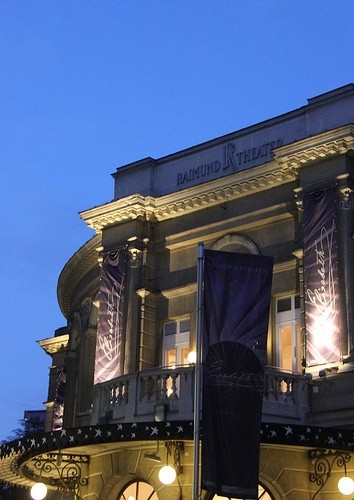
[145,441,162,464]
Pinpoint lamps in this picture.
[144,440,161,463]
[158,441,185,486]
[307,448,354,500]
[30,452,90,500]
